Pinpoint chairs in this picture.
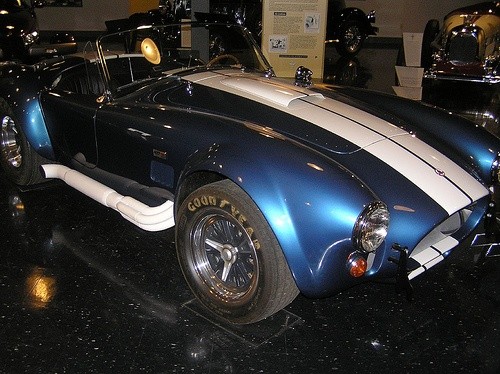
[56,56,94,96]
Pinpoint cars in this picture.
[417,1,500,81]
[1,0,46,55]
[105,0,380,67]
[0,20,500,326]
